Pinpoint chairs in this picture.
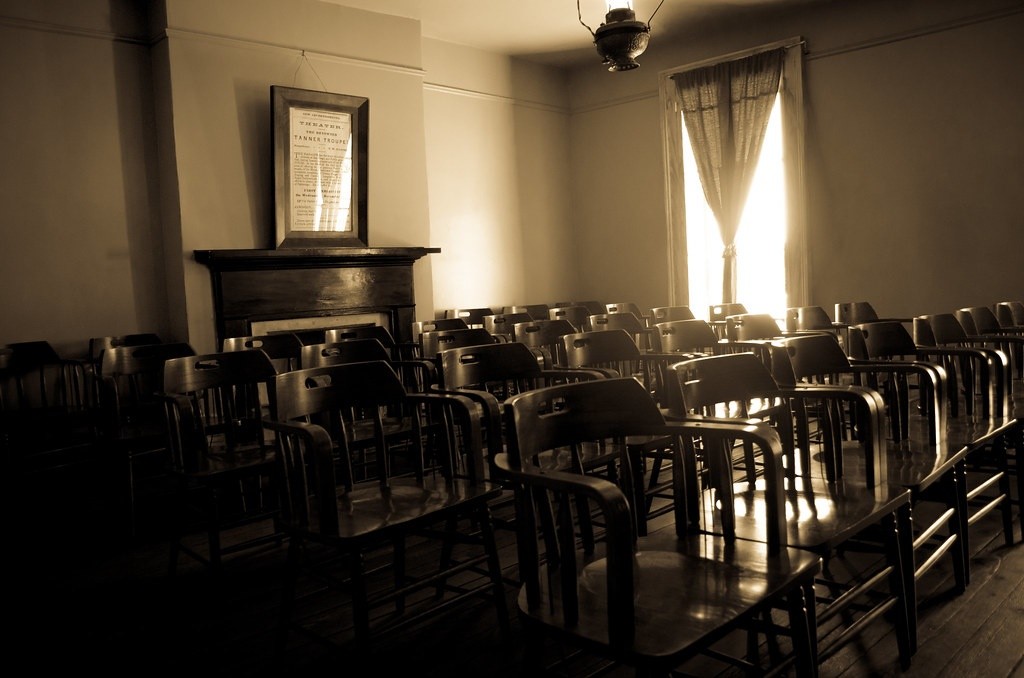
[0,301,1024,678]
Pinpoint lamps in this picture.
[577,0,664,71]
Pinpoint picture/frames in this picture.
[271,85,370,250]
